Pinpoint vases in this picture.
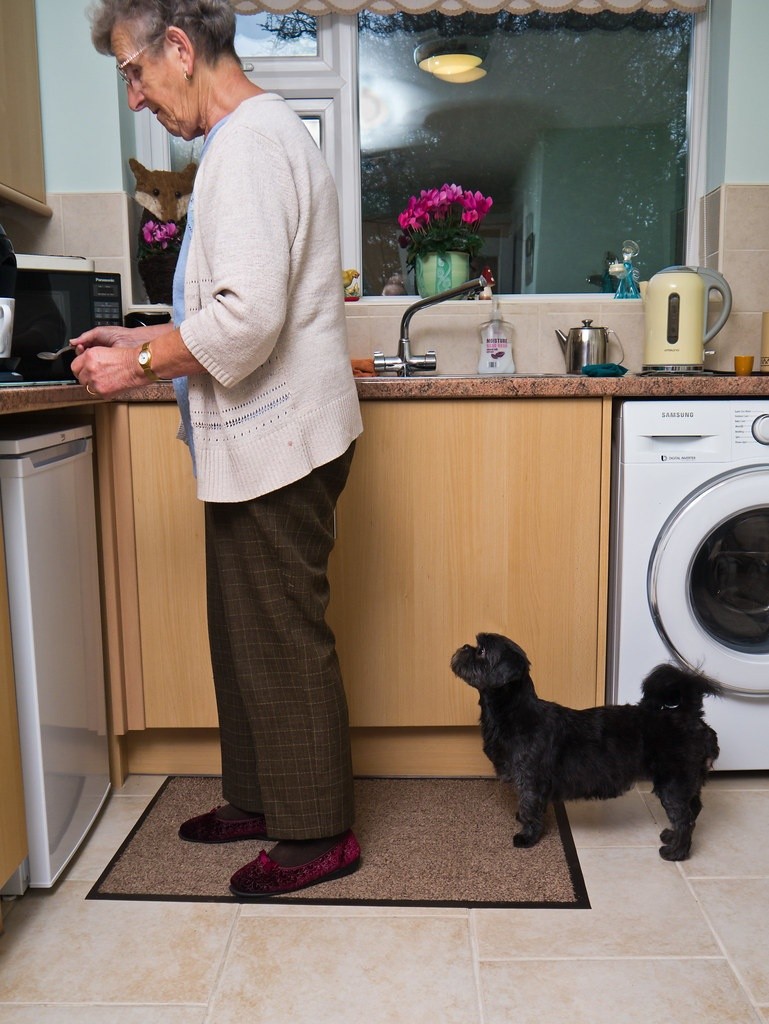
[416,251,470,300]
[138,254,180,306]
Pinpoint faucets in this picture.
[373,274,488,376]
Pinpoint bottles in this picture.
[476,298,516,375]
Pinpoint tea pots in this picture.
[554,319,624,375]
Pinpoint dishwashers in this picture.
[2,418,112,890]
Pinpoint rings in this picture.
[86,385,96,395]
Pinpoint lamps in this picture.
[413,36,495,83]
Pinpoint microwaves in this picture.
[16,253,129,382]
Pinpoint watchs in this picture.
[138,342,159,381]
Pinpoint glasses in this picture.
[116,33,166,86]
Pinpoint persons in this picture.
[66,0,364,899]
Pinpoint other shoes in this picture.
[229,830,362,896]
[178,804,264,844]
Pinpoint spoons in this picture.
[37,345,74,360]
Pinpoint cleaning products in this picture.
[476,294,516,374]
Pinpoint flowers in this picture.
[397,183,493,265]
[134,221,184,259]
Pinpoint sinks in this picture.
[371,372,577,378]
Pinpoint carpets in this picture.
[86,772,592,908]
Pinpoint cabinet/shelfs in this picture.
[94,394,612,788]
[0,0,54,216]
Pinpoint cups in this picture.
[734,356,754,377]
[0,297,15,358]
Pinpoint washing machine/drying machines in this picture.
[611,397,769,771]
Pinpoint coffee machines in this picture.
[0,224,24,382]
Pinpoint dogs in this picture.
[451,631,719,862]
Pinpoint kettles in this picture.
[638,265,732,373]
